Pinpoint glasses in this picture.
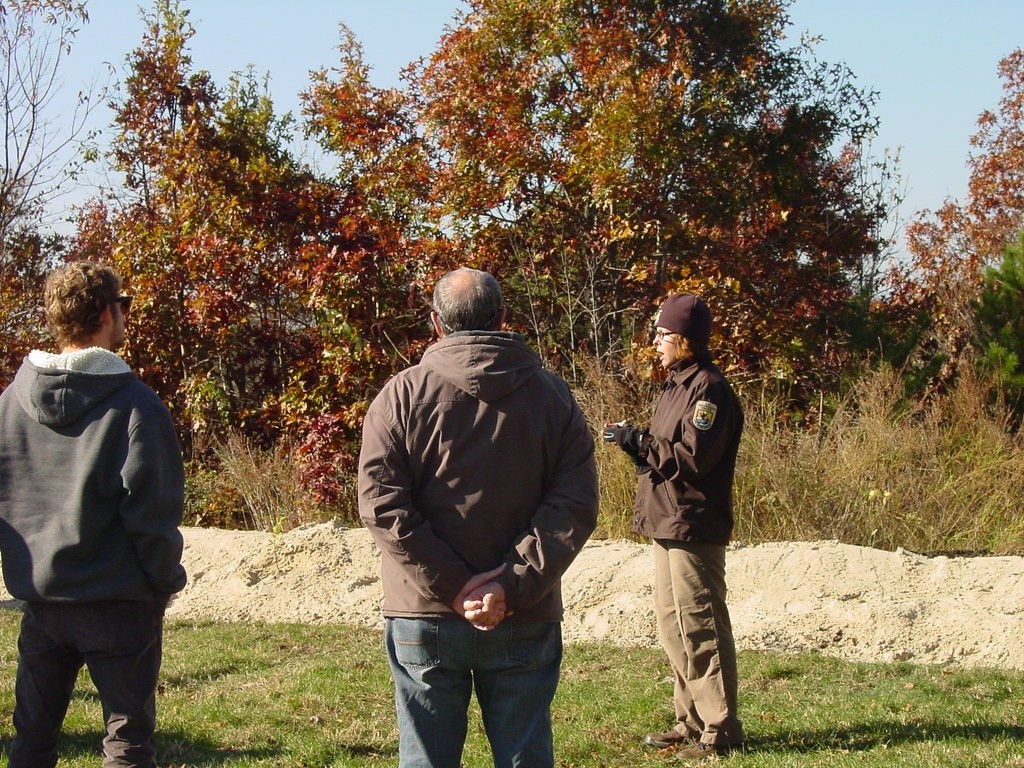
[99,295,133,315]
[655,330,677,340]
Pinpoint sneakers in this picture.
[678,742,749,758]
[645,731,693,748]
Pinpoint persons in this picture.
[1,262,188,768]
[356,269,599,768]
[601,295,747,757]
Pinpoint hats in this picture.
[654,293,712,344]
